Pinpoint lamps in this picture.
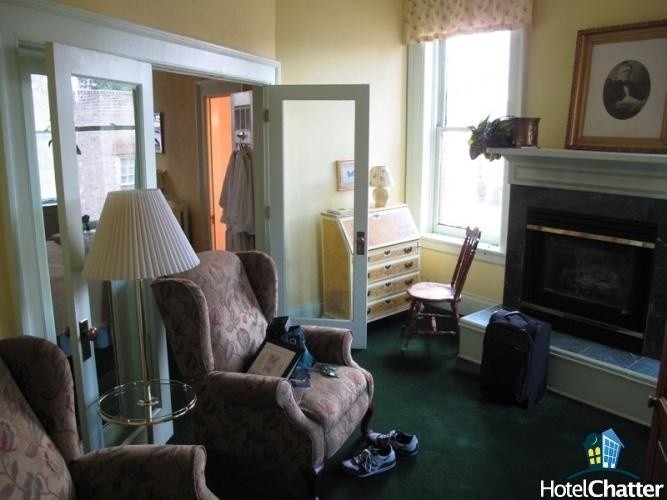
[369,165,390,209]
[80,189,200,410]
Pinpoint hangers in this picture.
[234,143,250,151]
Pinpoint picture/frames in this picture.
[154,112,164,154]
[564,20,667,154]
[336,159,356,191]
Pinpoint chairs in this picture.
[401,227,481,353]
[0,335,219,500]
[150,250,374,500]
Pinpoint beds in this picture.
[95,380,197,444]
[43,204,118,335]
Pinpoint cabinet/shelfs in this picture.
[319,200,420,325]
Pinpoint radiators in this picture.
[167,200,190,242]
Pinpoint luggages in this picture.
[479,308,552,410]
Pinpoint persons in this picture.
[603,60,646,120]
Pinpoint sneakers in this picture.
[337,444,397,479]
[365,428,420,457]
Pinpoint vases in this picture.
[508,117,541,147]
